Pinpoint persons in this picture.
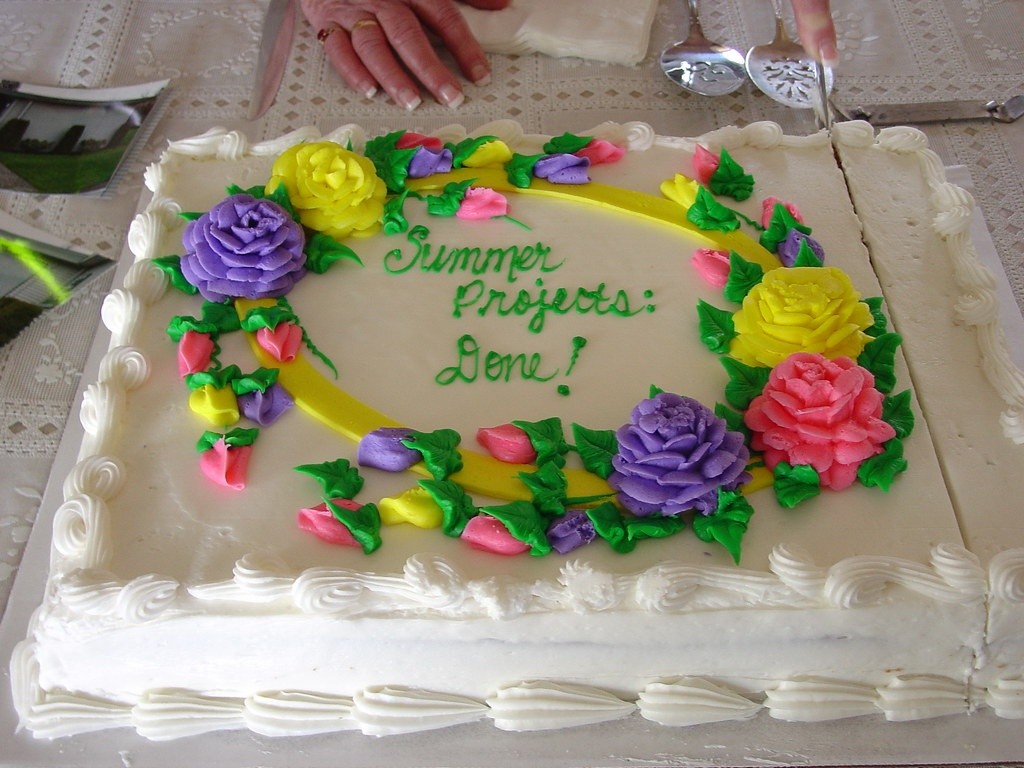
[299,0,838,111]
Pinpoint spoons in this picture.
[744,0,833,109]
[660,0,746,96]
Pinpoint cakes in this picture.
[9,116,1024,742]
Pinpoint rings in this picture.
[316,26,341,46]
[351,19,377,30]
